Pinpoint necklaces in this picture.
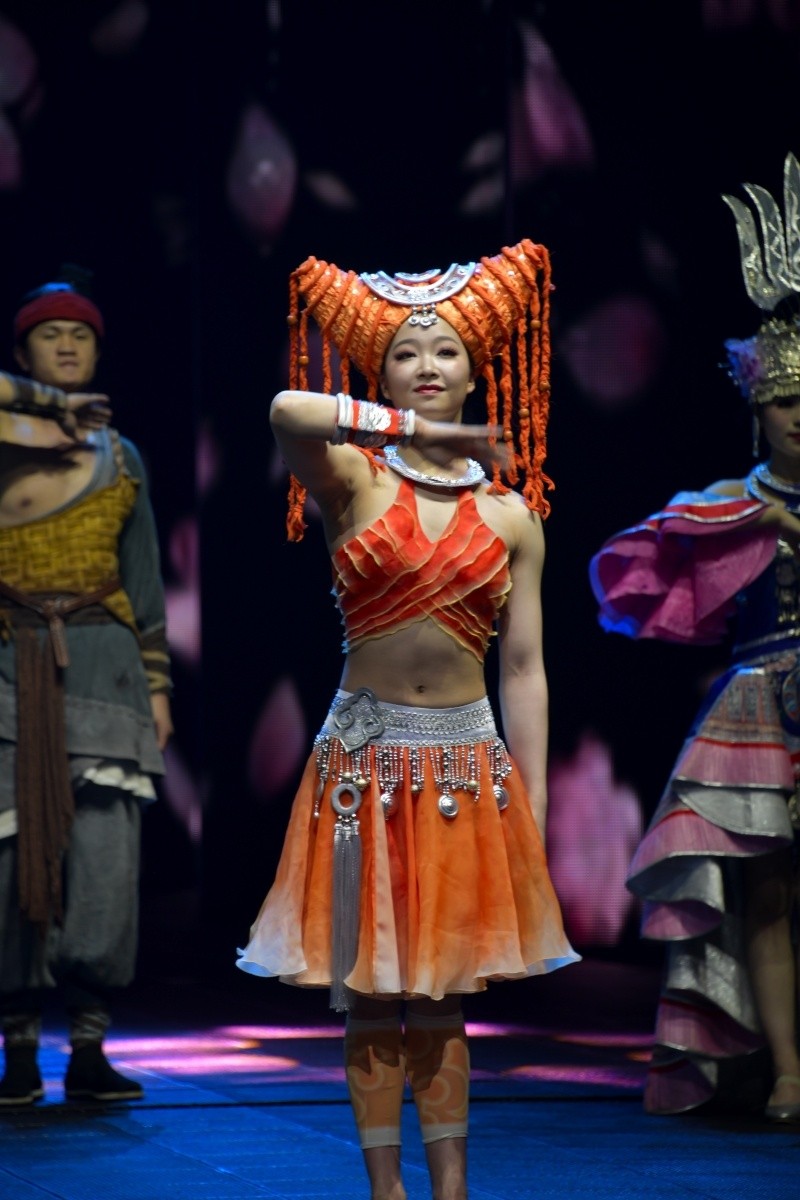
[747,464,800,515]
[385,446,485,487]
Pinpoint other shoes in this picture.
[0,1047,44,1105]
[65,1045,142,1100]
[768,1075,800,1123]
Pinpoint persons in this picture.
[0,283,172,1102]
[591,154,800,1124]
[235,240,583,1200]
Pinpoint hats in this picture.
[15,282,105,335]
